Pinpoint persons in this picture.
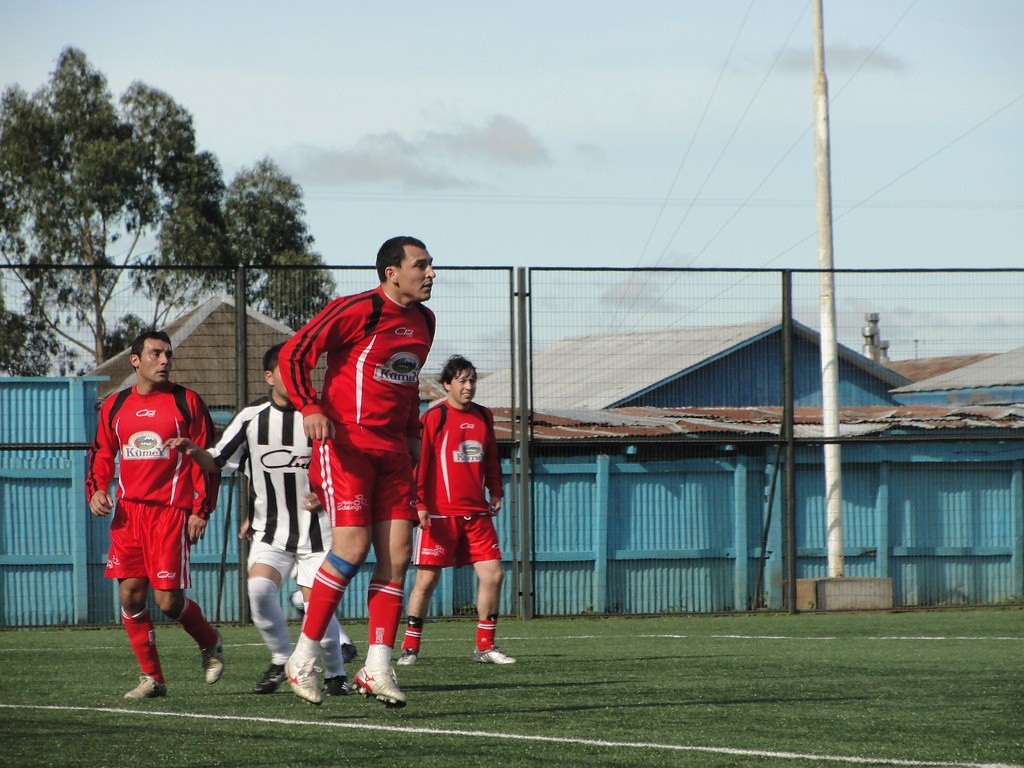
[278,236,437,709]
[395,357,517,667]
[236,449,361,665]
[164,341,352,697]
[83,330,224,700]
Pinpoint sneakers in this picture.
[284,660,324,706]
[472,644,517,666]
[396,648,419,666]
[351,667,408,709]
[200,629,225,685]
[340,640,358,664]
[123,671,167,700]
[253,662,287,695]
[324,675,349,697]
